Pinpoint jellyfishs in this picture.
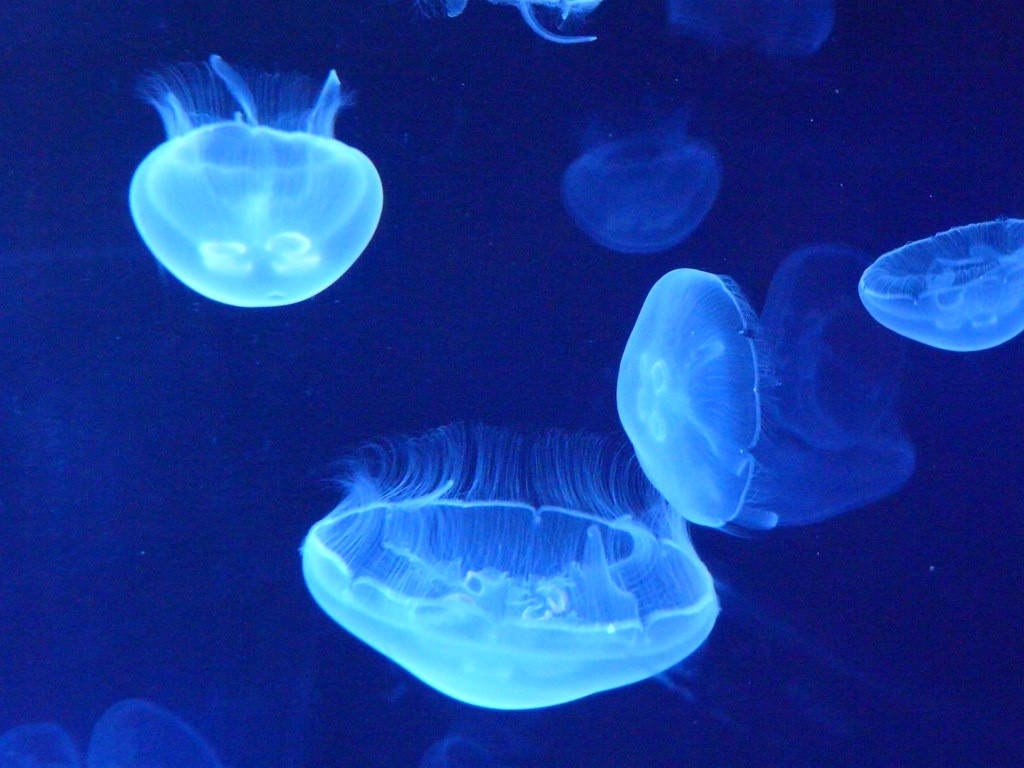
[0,2,1024,768]
